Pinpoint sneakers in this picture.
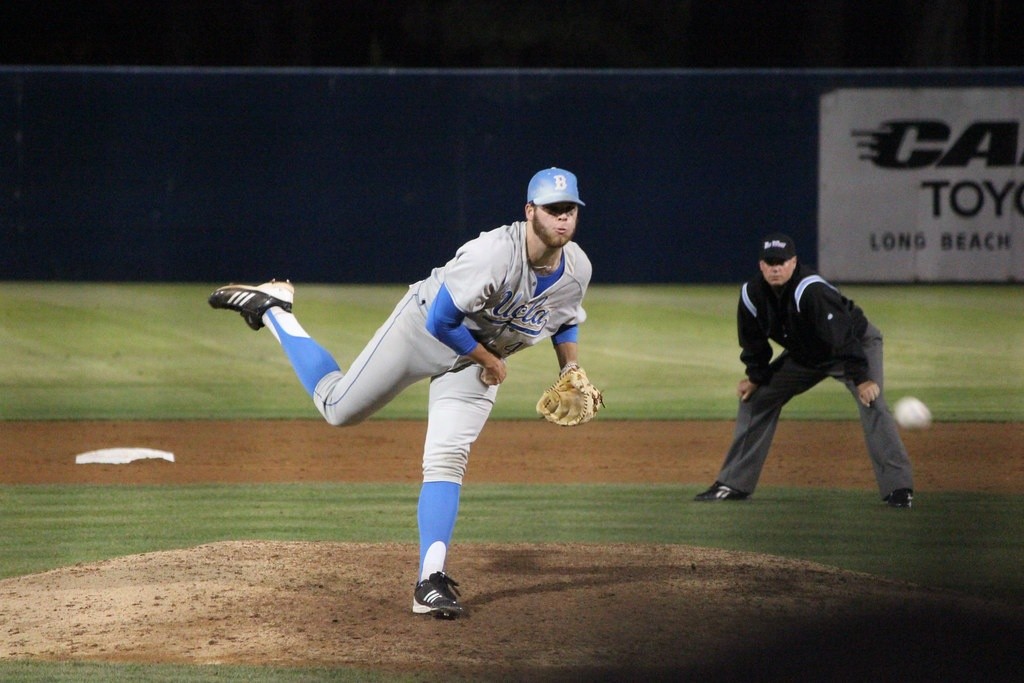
[411,572,463,617]
[693,480,749,501]
[207,278,295,330]
[887,488,914,509]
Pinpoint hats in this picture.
[526,167,585,207]
[758,232,796,260]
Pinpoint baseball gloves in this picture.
[535,365,608,430]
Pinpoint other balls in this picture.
[892,396,931,432]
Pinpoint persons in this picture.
[207,168,592,615]
[693,233,915,509]
